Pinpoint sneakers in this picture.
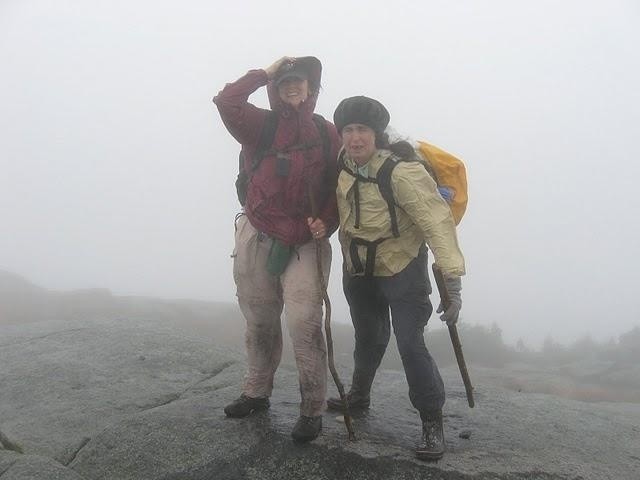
[223,390,272,419]
[290,409,324,440]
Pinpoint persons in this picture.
[327,96,466,461]
[212,56,342,443]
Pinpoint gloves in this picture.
[436,276,463,326]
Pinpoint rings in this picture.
[316,232,318,235]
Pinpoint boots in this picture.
[326,372,373,414]
[415,408,447,460]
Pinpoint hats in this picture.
[333,97,392,135]
[276,63,305,86]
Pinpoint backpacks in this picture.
[235,110,328,209]
[338,136,468,240]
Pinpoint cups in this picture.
[265,239,292,274]
